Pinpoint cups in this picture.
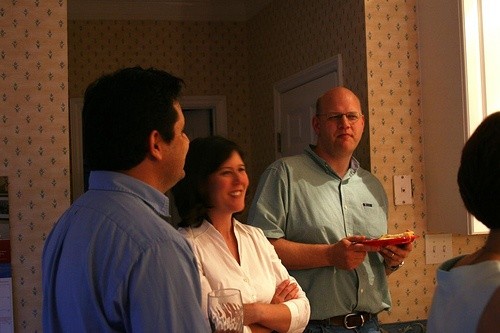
[208,288,244,332]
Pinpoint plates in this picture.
[353,235,419,252]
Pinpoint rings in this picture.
[389,253,394,258]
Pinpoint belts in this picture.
[307,312,378,330]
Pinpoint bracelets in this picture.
[384,259,405,269]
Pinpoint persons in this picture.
[42,67,213,333]
[247,88,413,333]
[170,134,311,333]
[426,112,500,333]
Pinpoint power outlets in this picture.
[426,232,455,264]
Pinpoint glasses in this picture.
[317,112,363,123]
